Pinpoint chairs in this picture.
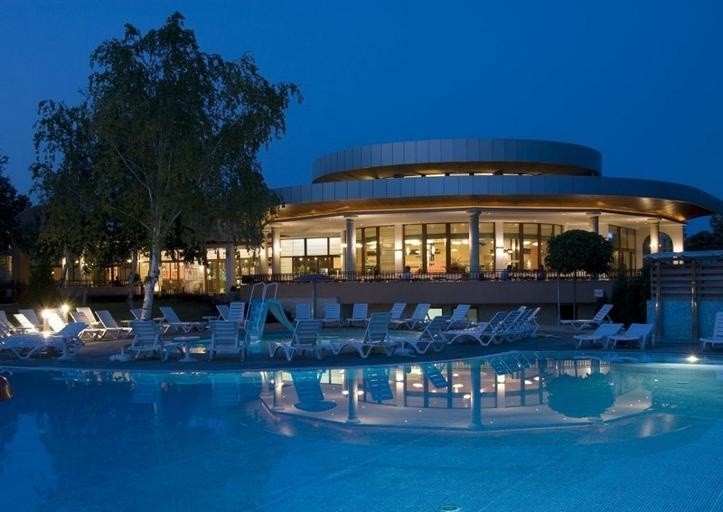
[0,301,248,362]
[484,353,536,375]
[361,368,394,399]
[208,371,241,406]
[698,311,723,350]
[291,369,323,400]
[126,370,162,403]
[268,299,542,358]
[417,362,447,389]
[560,304,656,352]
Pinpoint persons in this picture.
[500,265,512,280]
[536,264,543,279]
[113,277,121,287]
[401,266,412,279]
